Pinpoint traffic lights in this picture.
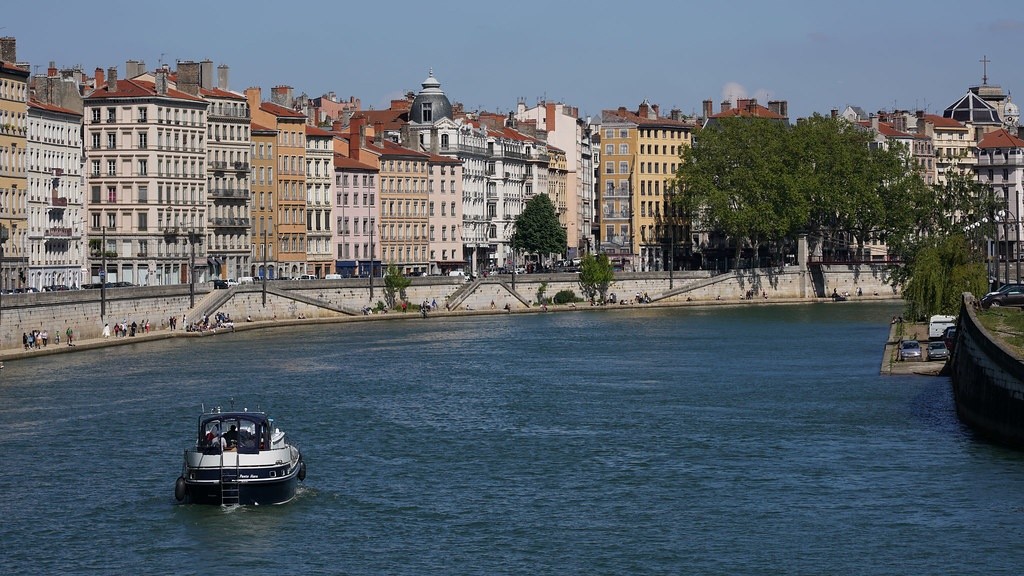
[19,270,23,279]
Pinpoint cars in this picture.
[925,341,950,362]
[400,264,527,277]
[207,273,369,289]
[898,340,923,362]
[0,281,135,295]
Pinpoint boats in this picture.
[174,395,307,507]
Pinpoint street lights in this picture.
[996,190,1020,283]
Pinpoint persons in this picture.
[856,287,862,296]
[504,303,511,312]
[572,302,575,307]
[66,326,73,344]
[893,315,897,323]
[423,271,427,277]
[103,311,235,340]
[402,302,407,314]
[491,300,494,309]
[226,425,238,439]
[56,330,60,343]
[298,313,305,319]
[589,293,614,306]
[23,329,48,351]
[465,271,487,282]
[540,303,547,312]
[620,291,653,306]
[383,305,387,314]
[419,299,438,318]
[740,289,754,300]
[246,315,254,322]
[361,307,373,315]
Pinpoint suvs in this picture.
[981,283,1024,311]
